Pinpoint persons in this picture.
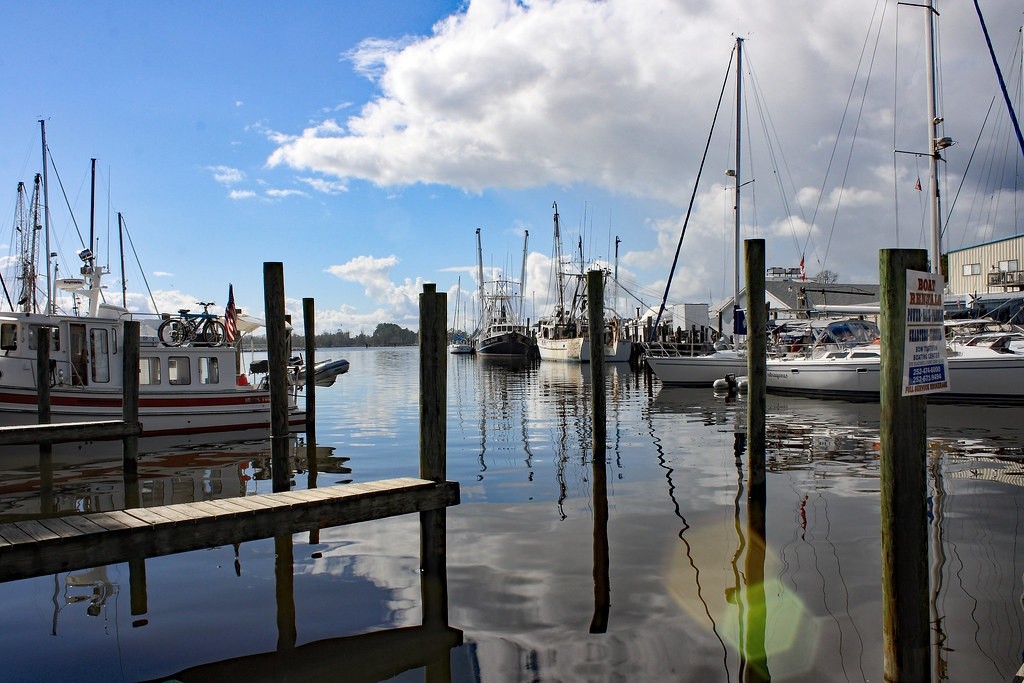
[989,265,995,284]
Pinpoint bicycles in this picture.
[158,302,226,348]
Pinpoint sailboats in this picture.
[645,3,1024,392]
[0,120,309,472]
[448,197,633,365]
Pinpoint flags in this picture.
[800,255,805,281]
[915,179,922,191]
[225,286,237,344]
